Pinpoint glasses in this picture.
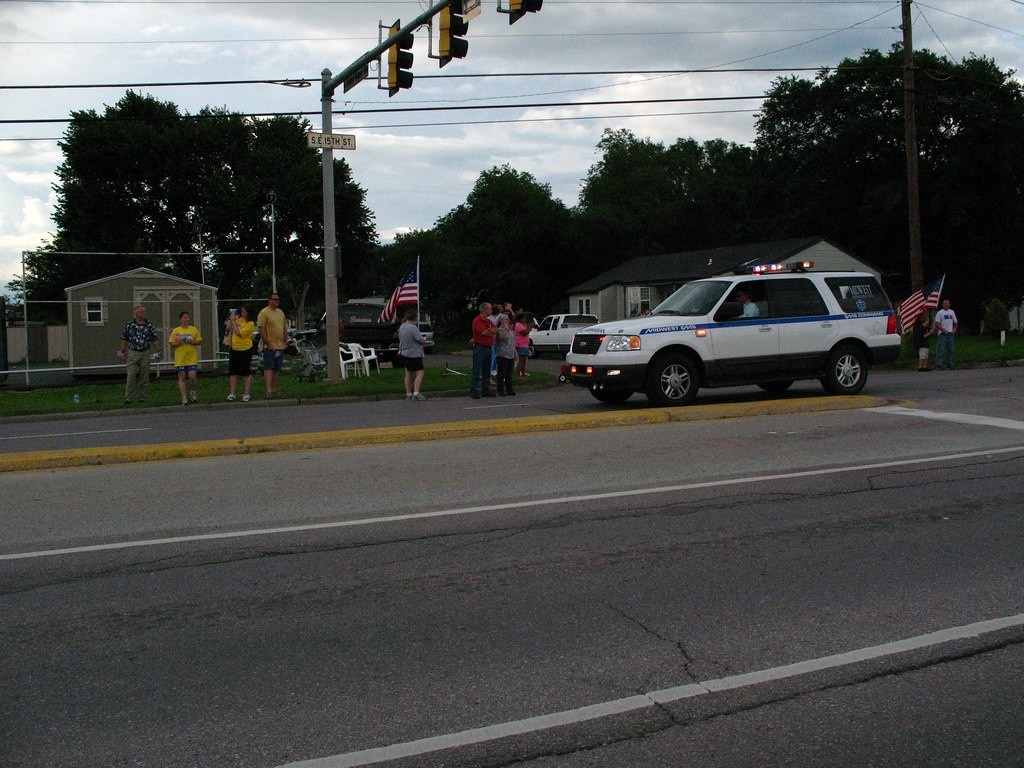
[272,298,280,302]
[736,295,743,297]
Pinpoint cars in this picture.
[413,321,435,354]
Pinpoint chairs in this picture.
[339,342,379,379]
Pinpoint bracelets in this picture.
[158,349,161,352]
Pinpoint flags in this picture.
[897,277,944,335]
[377,263,418,323]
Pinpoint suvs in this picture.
[527,312,601,361]
[564,260,903,409]
[321,301,406,369]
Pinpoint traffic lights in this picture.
[508,0,544,25]
[387,19,415,98]
[438,0,470,69]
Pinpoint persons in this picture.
[223,312,240,359]
[486,303,535,385]
[398,309,425,401]
[915,314,934,372]
[225,304,255,402]
[497,314,517,397]
[470,302,498,399]
[257,293,288,398]
[935,299,958,371]
[120,306,161,405]
[732,290,759,318]
[169,312,202,406]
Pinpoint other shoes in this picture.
[125,396,131,404]
[138,395,144,402]
[242,393,250,401]
[190,395,198,403]
[498,390,506,396]
[918,366,932,371]
[411,393,427,400]
[225,394,237,401]
[506,390,516,395]
[405,394,413,400]
[182,400,189,406]
[266,392,279,399]
[935,365,945,371]
[482,391,496,397]
[471,392,481,398]
